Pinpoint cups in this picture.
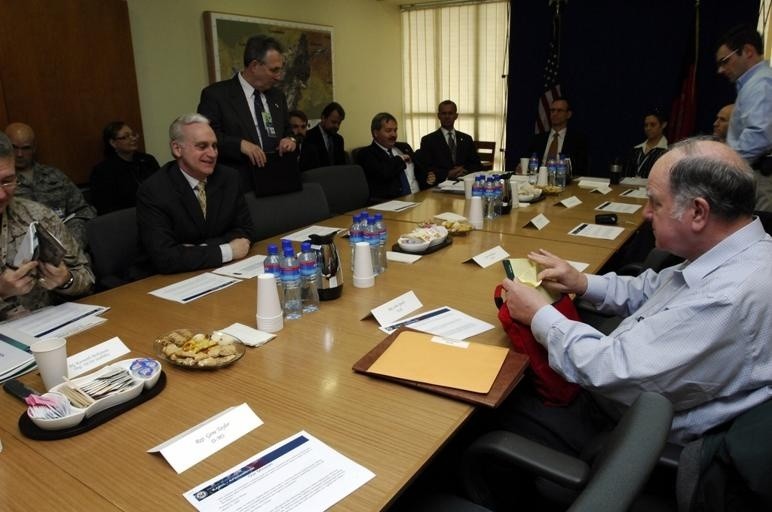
[256,242,376,333]
[30,337,69,392]
[464,158,620,230]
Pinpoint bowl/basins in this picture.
[397,235,432,252]
[414,225,448,245]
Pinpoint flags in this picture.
[667,67,696,145]
[534,7,560,136]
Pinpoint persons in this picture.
[0,134,94,322]
[1,122,162,253]
[358,112,426,203]
[624,105,736,177]
[531,96,580,177]
[136,113,255,282]
[289,99,347,171]
[715,28,771,233]
[473,135,772,470]
[196,35,300,190]
[421,100,483,187]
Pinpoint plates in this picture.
[152,327,246,370]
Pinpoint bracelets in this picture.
[289,136,296,143]
[60,275,74,290]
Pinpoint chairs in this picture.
[301,164,371,216]
[437,391,684,511]
[242,182,332,237]
[474,141,496,171]
[616,243,672,276]
[81,208,138,290]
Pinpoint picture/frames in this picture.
[201,10,338,123]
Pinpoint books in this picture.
[0,332,33,385]
[12,221,67,267]
[352,326,530,409]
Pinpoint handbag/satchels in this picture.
[494,284,581,409]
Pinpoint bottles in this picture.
[264,211,387,319]
[472,152,568,216]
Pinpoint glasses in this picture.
[550,108,568,114]
[0,176,22,192]
[716,49,742,69]
[114,133,140,142]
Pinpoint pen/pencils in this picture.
[5,262,47,284]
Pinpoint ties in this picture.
[253,88,270,156]
[448,132,457,168]
[196,182,207,221]
[546,134,560,168]
[388,148,394,158]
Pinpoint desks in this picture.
[0,168,657,512]
